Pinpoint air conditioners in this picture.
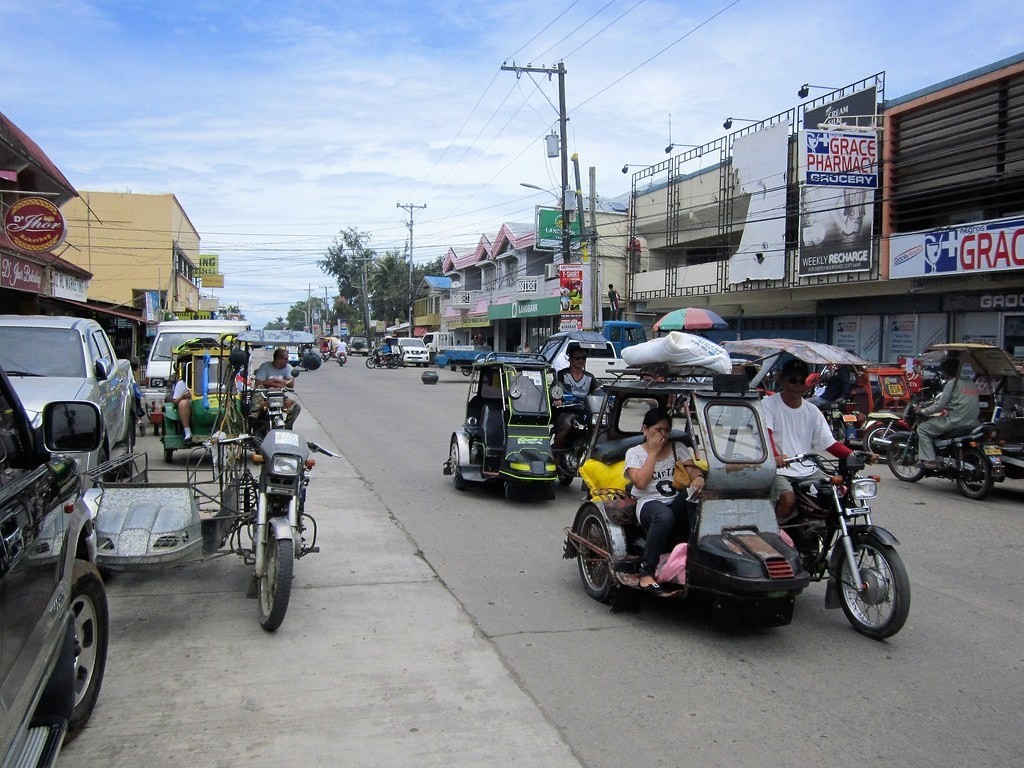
[545,264,557,278]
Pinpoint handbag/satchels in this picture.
[671,440,691,490]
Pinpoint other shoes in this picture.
[916,463,937,468]
[638,580,662,594]
[183,437,192,444]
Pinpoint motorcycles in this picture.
[365,346,401,369]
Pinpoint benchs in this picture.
[578,427,708,572]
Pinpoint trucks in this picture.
[422,331,492,365]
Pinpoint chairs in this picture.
[464,403,509,458]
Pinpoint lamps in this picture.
[723,117,758,130]
[798,83,838,99]
[665,143,698,154]
[622,164,651,173]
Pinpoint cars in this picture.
[0,313,138,767]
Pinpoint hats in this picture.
[941,357,960,377]
[781,360,810,376]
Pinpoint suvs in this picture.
[398,337,430,367]
[347,336,369,356]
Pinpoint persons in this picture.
[483,340,488,346]
[749,358,881,521]
[335,339,349,360]
[912,357,980,470]
[608,284,621,321]
[172,366,193,442]
[523,343,530,352]
[548,346,600,450]
[249,349,302,430]
[321,340,331,352]
[622,409,705,591]
[455,340,462,346]
[561,291,571,310]
[380,340,390,364]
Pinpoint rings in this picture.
[699,490,702,493]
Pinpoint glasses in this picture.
[571,355,587,360]
[785,376,805,385]
[280,357,288,360]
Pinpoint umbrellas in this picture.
[653,307,729,332]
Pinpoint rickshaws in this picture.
[810,343,1024,499]
[563,361,911,641]
[443,322,872,508]
[81,319,346,632]
